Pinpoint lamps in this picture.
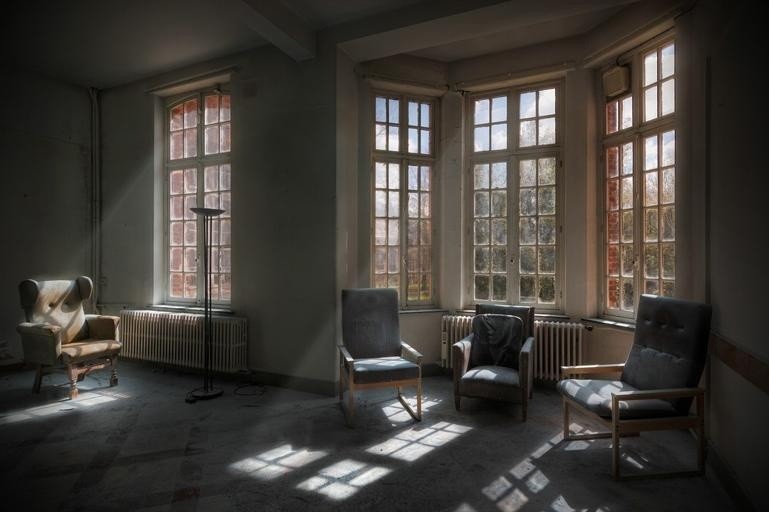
[184,207,226,404]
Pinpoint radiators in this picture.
[441,314,585,382]
[117,310,248,377]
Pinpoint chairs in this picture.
[336,287,424,427]
[556,293,713,482]
[16,276,122,400]
[451,303,535,417]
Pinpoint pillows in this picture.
[470,314,524,371]
